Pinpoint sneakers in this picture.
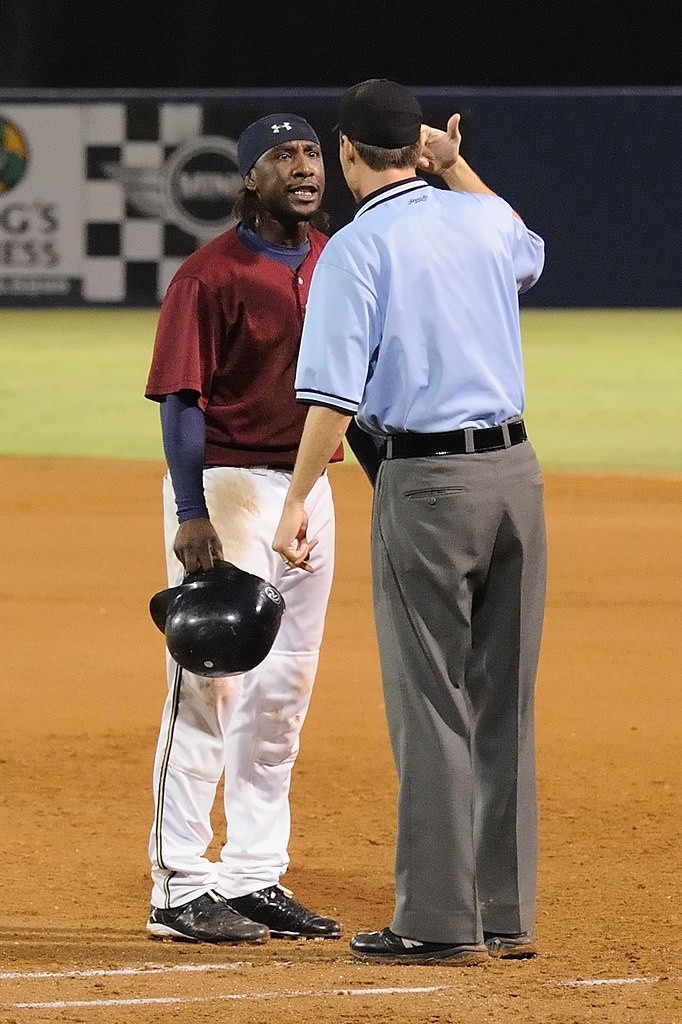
[227,884,345,941]
[483,928,535,958]
[146,889,270,945]
[349,927,489,967]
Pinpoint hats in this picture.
[332,78,423,149]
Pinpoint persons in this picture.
[273,79,546,967]
[146,113,345,946]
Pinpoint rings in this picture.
[284,559,290,564]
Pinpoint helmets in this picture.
[149,559,286,677]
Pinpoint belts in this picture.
[380,419,528,460]
[267,464,326,476]
